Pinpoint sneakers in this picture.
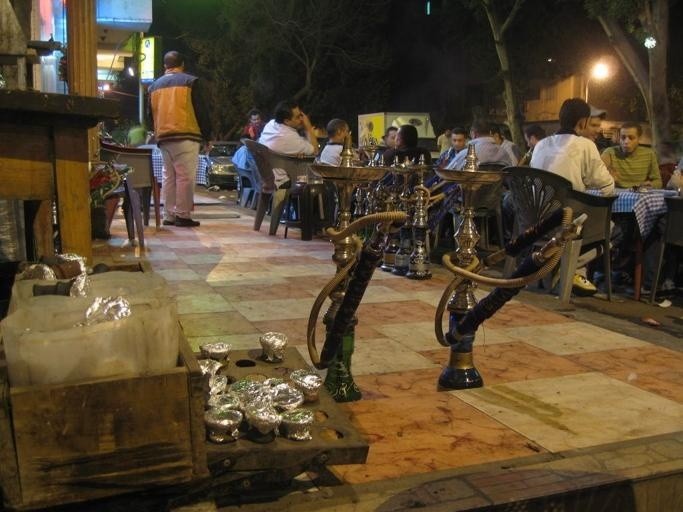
[572,270,678,298]
[163,220,176,224]
[175,215,200,226]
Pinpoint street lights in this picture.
[585,57,617,104]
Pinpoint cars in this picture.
[200,139,243,190]
[462,117,512,142]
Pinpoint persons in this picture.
[238,99,361,220]
[531,97,615,296]
[144,50,211,228]
[126,121,144,146]
[582,103,683,190]
[376,125,547,196]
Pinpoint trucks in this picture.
[355,111,441,160]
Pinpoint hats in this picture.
[588,104,606,118]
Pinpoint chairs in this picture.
[100,139,160,228]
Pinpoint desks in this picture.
[0,89,121,268]
